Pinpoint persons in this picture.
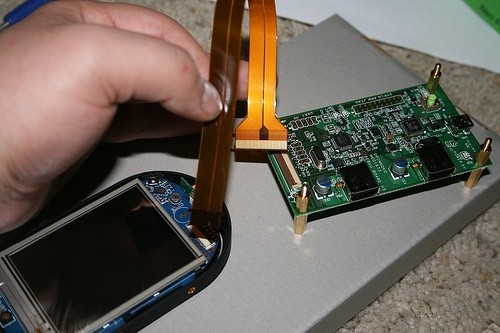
[0,0,279,235]
